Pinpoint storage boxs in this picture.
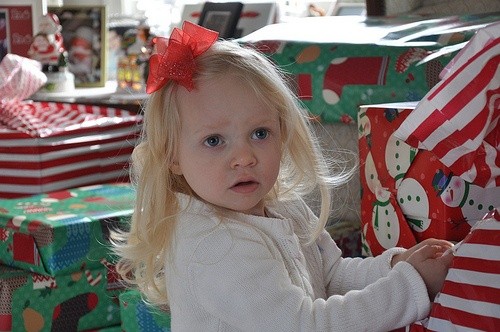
[356,97,474,259]
[231,14,500,124]
[1,114,171,332]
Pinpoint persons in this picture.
[26,13,69,73]
[109,21,454,332]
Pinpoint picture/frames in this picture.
[44,4,108,88]
[197,2,244,39]
